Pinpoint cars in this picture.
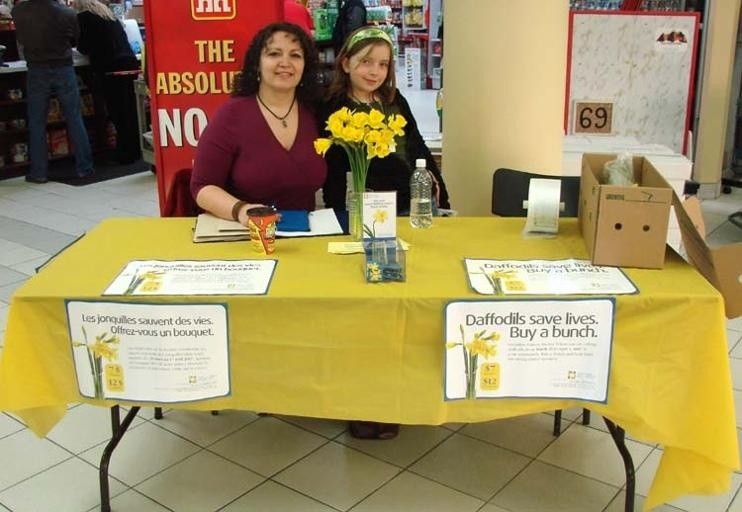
[247,207,278,257]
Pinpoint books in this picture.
[578,153,742,320]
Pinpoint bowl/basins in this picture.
[355,91,389,125]
[258,95,296,126]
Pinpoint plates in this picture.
[300,1,444,99]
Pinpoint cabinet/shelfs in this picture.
[409,158,433,229]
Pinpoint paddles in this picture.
[48,143,152,188]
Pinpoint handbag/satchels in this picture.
[231,198,247,221]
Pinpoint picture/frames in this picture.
[11,219,727,512]
[0,52,141,180]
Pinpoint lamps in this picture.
[489,166,581,439]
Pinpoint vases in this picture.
[314,107,406,233]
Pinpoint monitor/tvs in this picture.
[193,208,345,245]
[274,208,313,232]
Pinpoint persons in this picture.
[330,1,367,57]
[12,0,95,185]
[187,24,327,229]
[314,26,451,216]
[72,0,142,165]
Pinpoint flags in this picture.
[347,189,375,238]
